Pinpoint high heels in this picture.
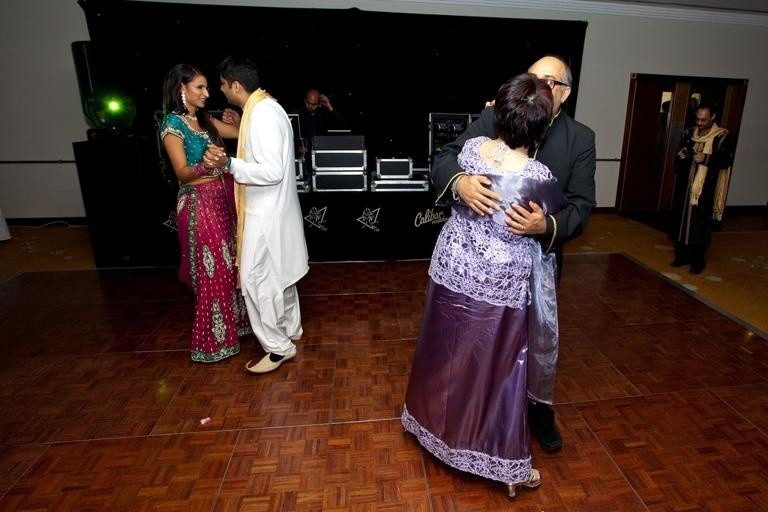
[503,471,542,499]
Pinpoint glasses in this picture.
[537,76,568,88]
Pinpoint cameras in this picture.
[682,149,695,159]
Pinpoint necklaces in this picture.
[183,113,197,120]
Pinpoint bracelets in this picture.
[192,161,209,178]
[453,175,466,200]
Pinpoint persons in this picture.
[300,89,336,136]
[202,61,309,373]
[669,107,734,274]
[431,56,596,454]
[161,64,254,363]
[402,72,558,498]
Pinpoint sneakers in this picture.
[524,403,564,454]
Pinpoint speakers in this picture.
[71,135,181,267]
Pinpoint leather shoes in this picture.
[245,343,297,374]
[669,253,708,274]
